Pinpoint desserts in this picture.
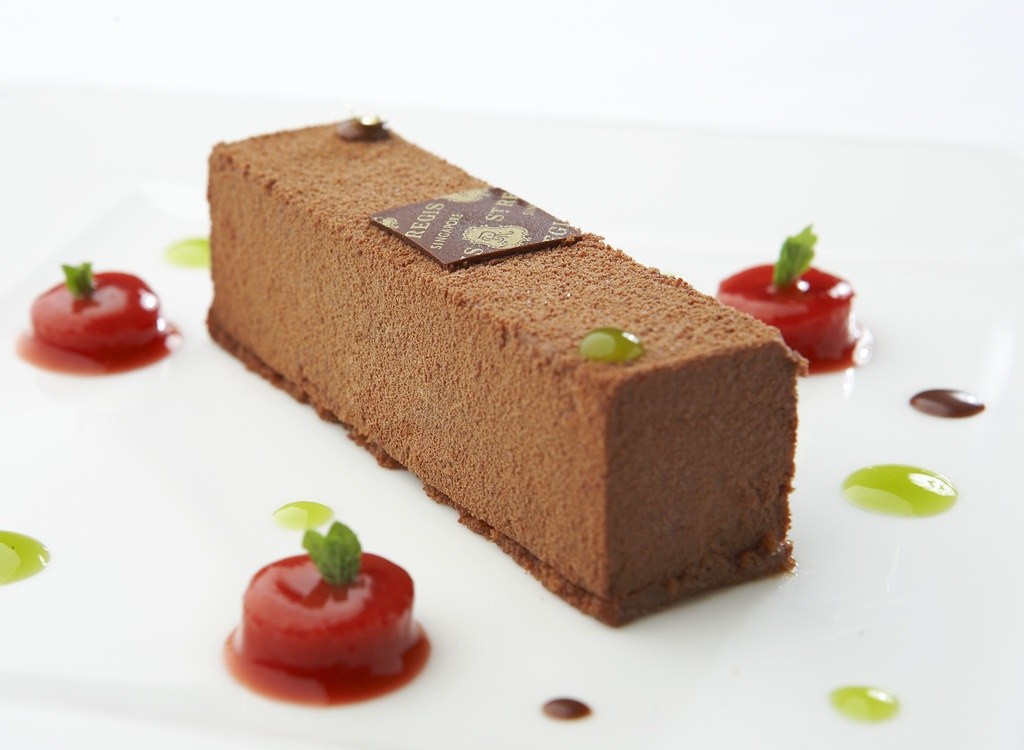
[205,115,813,629]
